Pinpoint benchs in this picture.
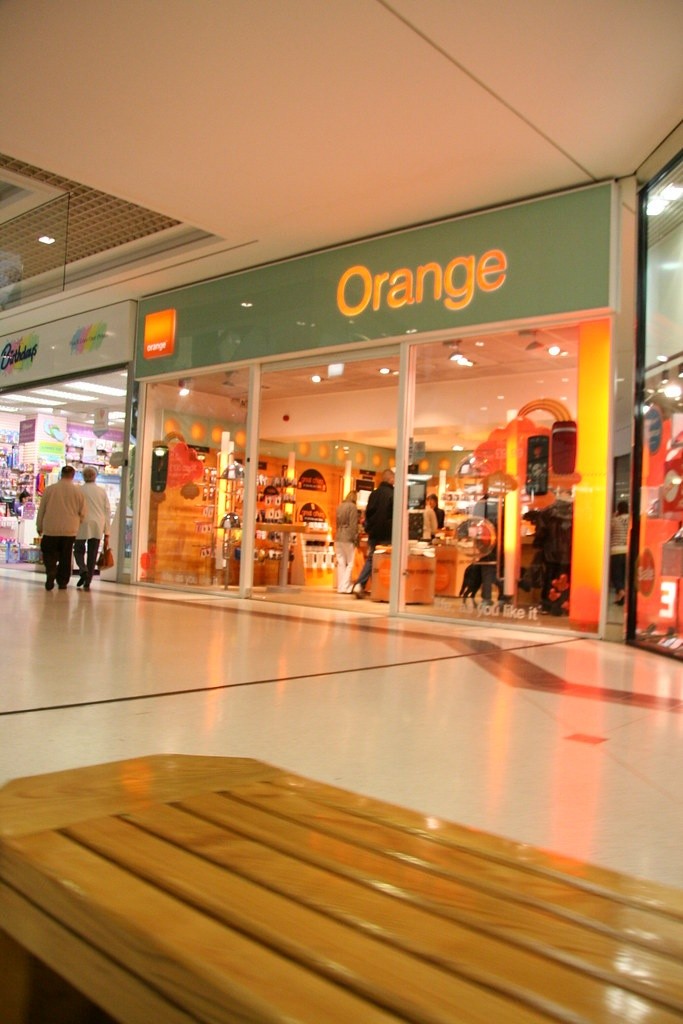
[0,756,683,1024]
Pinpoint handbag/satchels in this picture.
[99,547,114,572]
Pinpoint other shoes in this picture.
[354,585,364,599]
[45,571,68,590]
[77,572,90,590]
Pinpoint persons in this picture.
[473,495,503,606]
[36,466,112,591]
[335,469,394,599]
[408,494,445,545]
[610,501,630,606]
[14,491,30,516]
[520,487,573,617]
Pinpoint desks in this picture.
[257,523,310,587]
